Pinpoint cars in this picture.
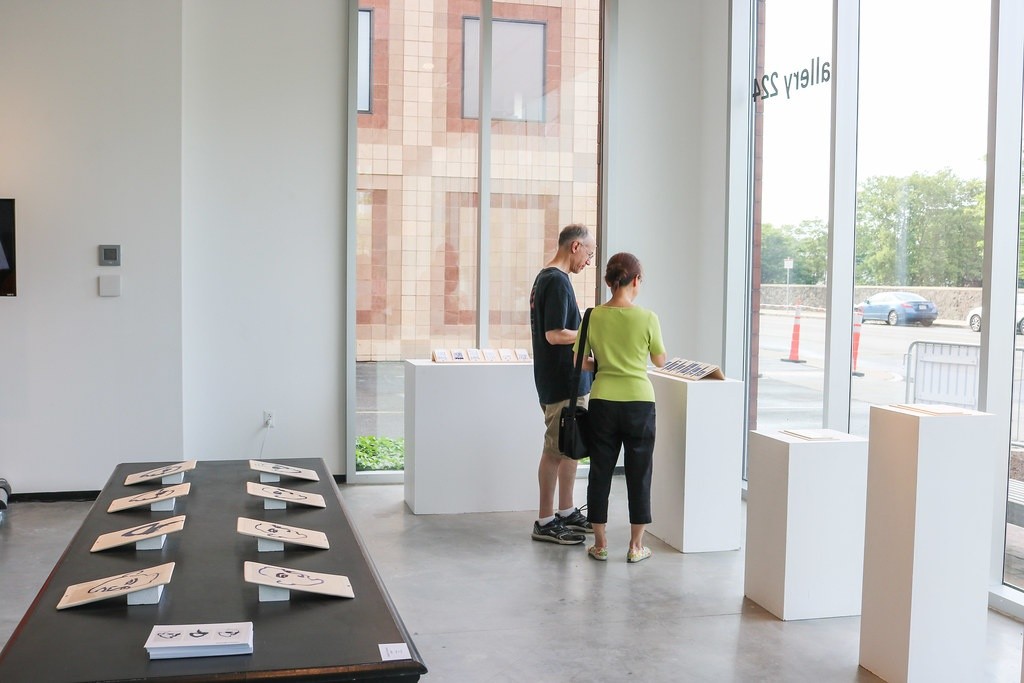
[966,290,1024,335]
[855,292,938,327]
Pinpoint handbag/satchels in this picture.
[557,406,592,459]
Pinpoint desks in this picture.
[1,457,428,682]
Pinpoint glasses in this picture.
[571,240,594,260]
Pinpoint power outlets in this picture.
[265,412,275,427]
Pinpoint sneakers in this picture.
[532,517,586,545]
[555,504,606,532]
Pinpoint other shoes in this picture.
[627,546,651,562]
[587,545,608,560]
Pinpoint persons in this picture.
[572,253,666,563]
[530,223,595,545]
